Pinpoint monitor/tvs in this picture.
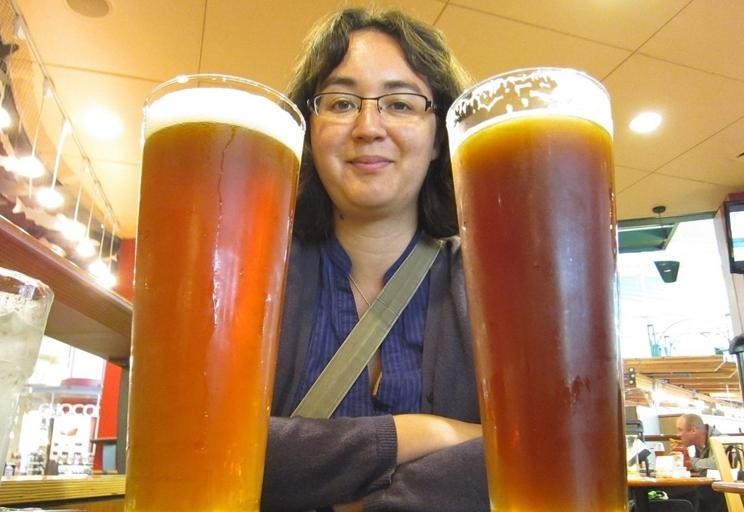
[724,200,744,273]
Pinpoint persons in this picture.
[670,414,727,512]
[260,7,491,512]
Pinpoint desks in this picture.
[630,472,712,511]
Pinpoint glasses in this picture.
[303,92,435,119]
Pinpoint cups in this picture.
[445,66,632,510]
[0,267,53,484]
[124,75,306,512]
